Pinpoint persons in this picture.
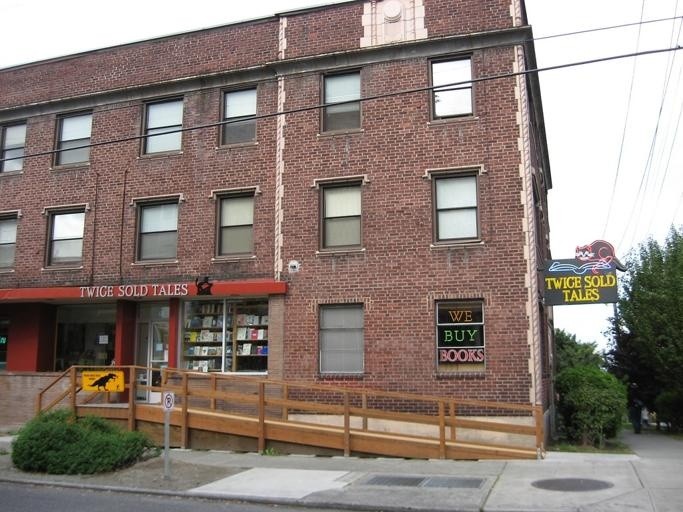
[627,383,644,434]
[641,407,649,430]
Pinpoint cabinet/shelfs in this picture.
[183,301,269,372]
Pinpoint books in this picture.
[183,303,268,372]
